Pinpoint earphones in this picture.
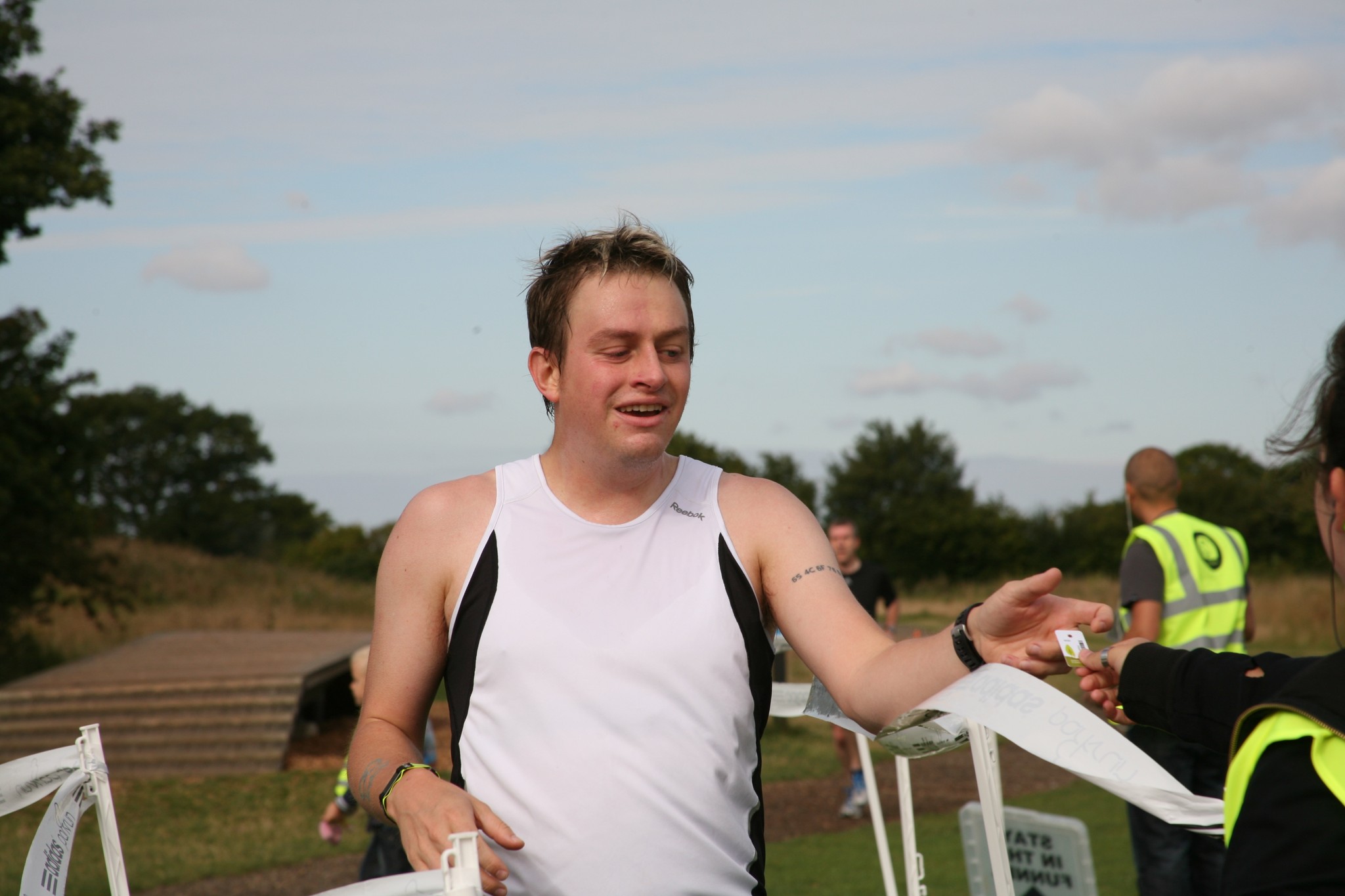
[1327,495,1339,524]
